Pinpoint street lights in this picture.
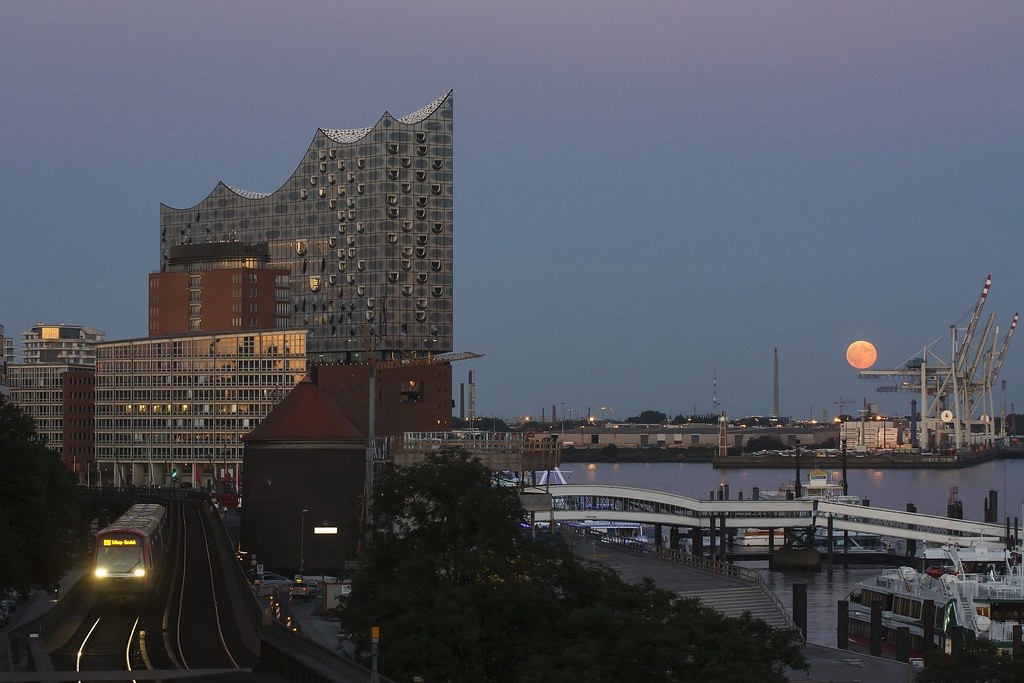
[298,509,308,575]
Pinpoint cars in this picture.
[288,579,312,600]
[254,573,294,597]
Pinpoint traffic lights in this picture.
[172,469,176,482]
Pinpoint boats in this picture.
[718,464,897,557]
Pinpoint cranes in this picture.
[856,272,1018,451]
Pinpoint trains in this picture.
[89,504,170,613]
